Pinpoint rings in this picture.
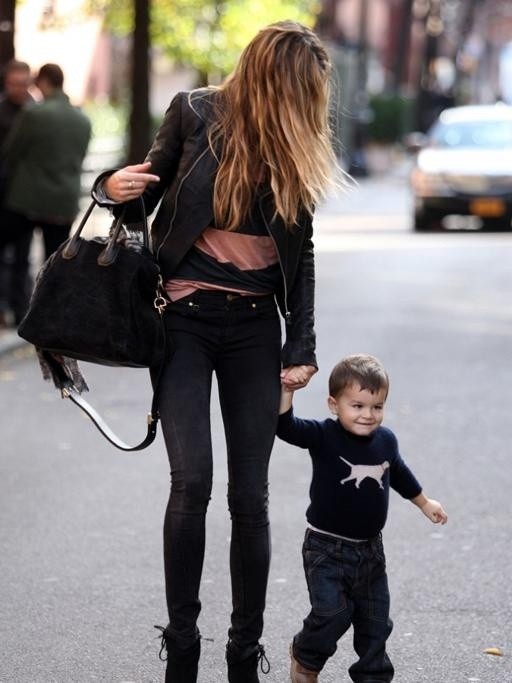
[128,181,134,190]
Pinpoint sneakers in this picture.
[289,640,319,683]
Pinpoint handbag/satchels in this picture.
[21,232,164,370]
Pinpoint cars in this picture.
[401,101,512,232]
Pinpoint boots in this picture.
[225,636,266,683]
[158,626,201,683]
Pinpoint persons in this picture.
[274,353,449,683]
[87,18,358,683]
[1,62,95,329]
[0,60,39,147]
